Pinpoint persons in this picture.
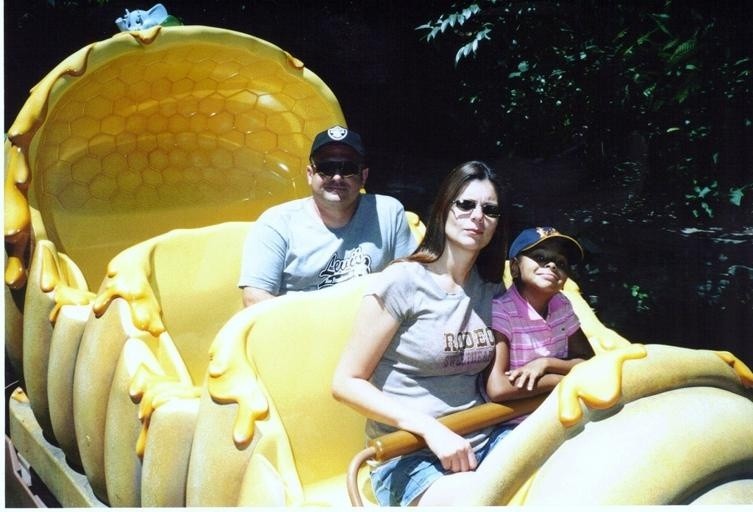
[484,226,598,432]
[331,160,511,506]
[237,126,418,309]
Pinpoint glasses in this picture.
[448,198,503,219]
[312,158,361,177]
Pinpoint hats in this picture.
[309,125,365,160]
[508,225,585,266]
